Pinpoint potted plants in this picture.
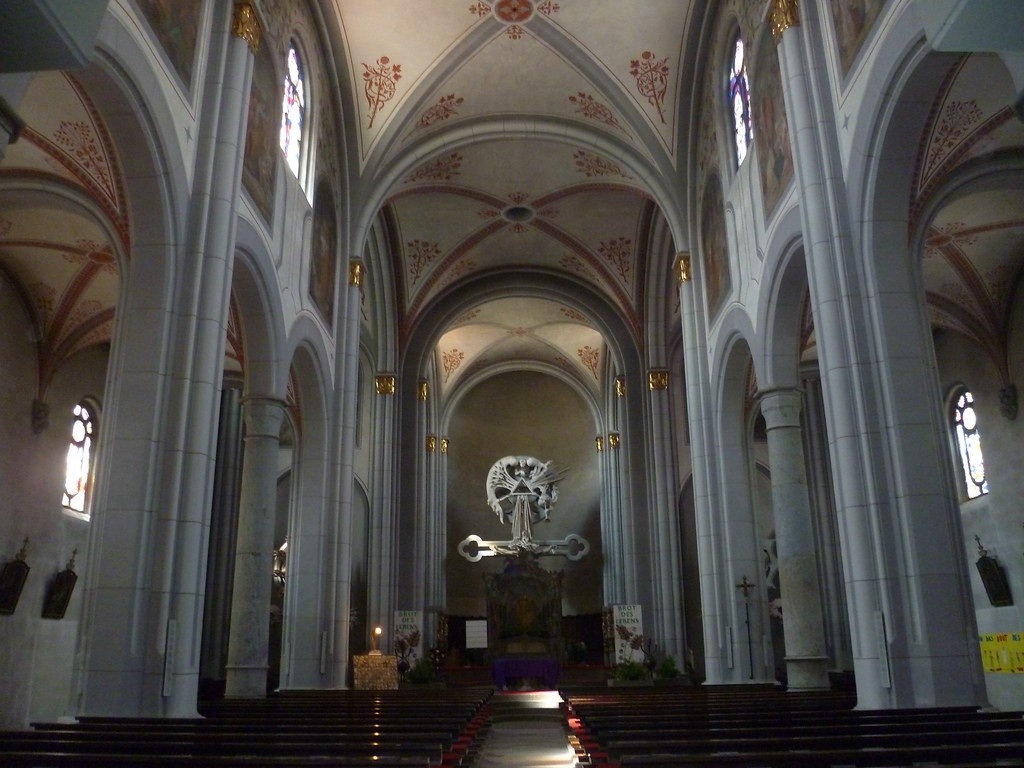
[404,658,436,690]
[680,661,706,686]
[568,643,591,665]
[612,660,649,687]
[653,656,682,687]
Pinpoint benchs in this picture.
[557,682,1024,768]
[0,682,499,768]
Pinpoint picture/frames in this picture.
[41,568,80,619]
[0,559,31,616]
[975,556,1013,607]
